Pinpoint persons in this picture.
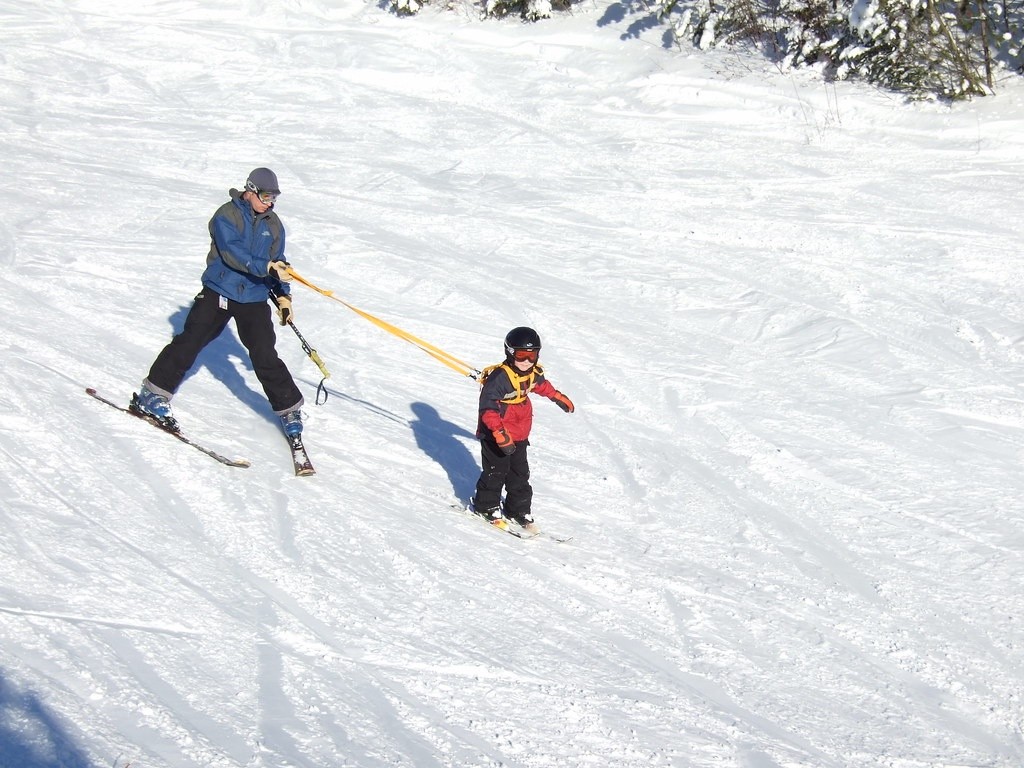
[128,167,304,449]
[465,326,574,535]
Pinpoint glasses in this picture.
[505,343,540,364]
[247,181,279,205]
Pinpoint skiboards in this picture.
[445,489,573,544]
[84,385,316,477]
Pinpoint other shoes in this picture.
[140,386,174,420]
[470,496,506,525]
[280,410,304,439]
[502,504,535,530]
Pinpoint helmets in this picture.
[244,167,284,196]
[504,326,542,356]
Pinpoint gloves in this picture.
[275,296,296,327]
[490,426,518,456]
[549,390,575,414]
[268,260,295,284]
